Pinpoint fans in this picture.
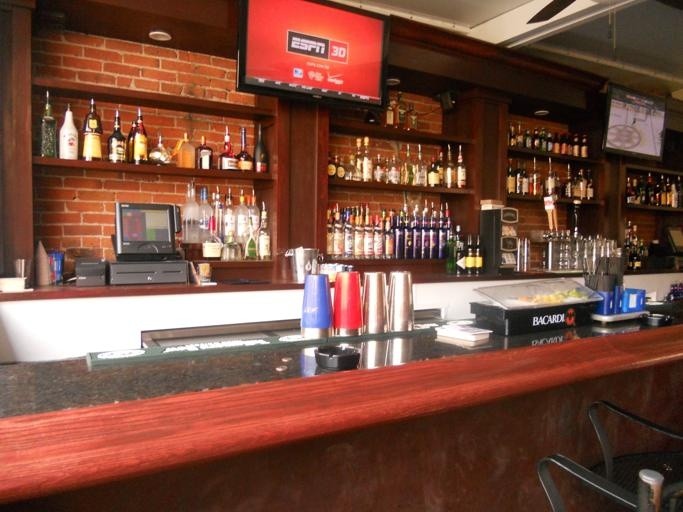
[515,0,620,23]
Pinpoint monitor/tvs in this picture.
[116,201,177,261]
[236,0,390,113]
[601,82,667,163]
[665,227,683,253]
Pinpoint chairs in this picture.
[534,400,683,512]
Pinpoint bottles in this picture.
[329,269,365,340]
[384,269,417,334]
[665,281,683,306]
[300,273,333,340]
[178,179,273,262]
[297,337,420,378]
[360,270,389,336]
[592,286,648,316]
[501,116,682,274]
[323,89,485,278]
[35,89,269,176]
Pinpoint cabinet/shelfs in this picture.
[484,46,618,275]
[0,0,302,294]
[614,85,683,275]
[302,3,496,275]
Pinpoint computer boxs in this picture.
[110,260,188,284]
[650,255,683,273]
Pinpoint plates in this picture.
[195,279,218,289]
[1,285,34,294]
[94,346,144,360]
[278,335,301,342]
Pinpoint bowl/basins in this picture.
[0,276,30,289]
[637,312,674,328]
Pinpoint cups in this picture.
[191,264,215,282]
[45,250,65,287]
[13,258,31,289]
